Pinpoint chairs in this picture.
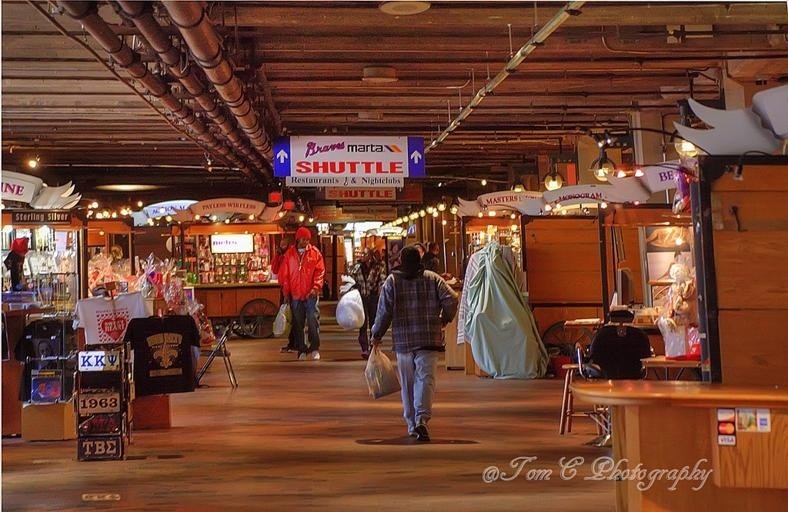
[198,320,239,388]
[575,343,611,446]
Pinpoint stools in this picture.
[559,365,586,434]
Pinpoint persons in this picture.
[278,227,325,361]
[341,240,458,440]
[272,240,299,353]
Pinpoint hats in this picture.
[295,227,311,242]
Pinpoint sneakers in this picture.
[311,350,320,361]
[280,344,297,353]
[297,350,308,360]
[407,417,431,442]
[361,348,370,357]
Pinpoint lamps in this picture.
[511,70,701,192]
[408,196,458,220]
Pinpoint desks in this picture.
[640,354,702,380]
[562,318,664,357]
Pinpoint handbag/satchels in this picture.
[272,302,291,339]
